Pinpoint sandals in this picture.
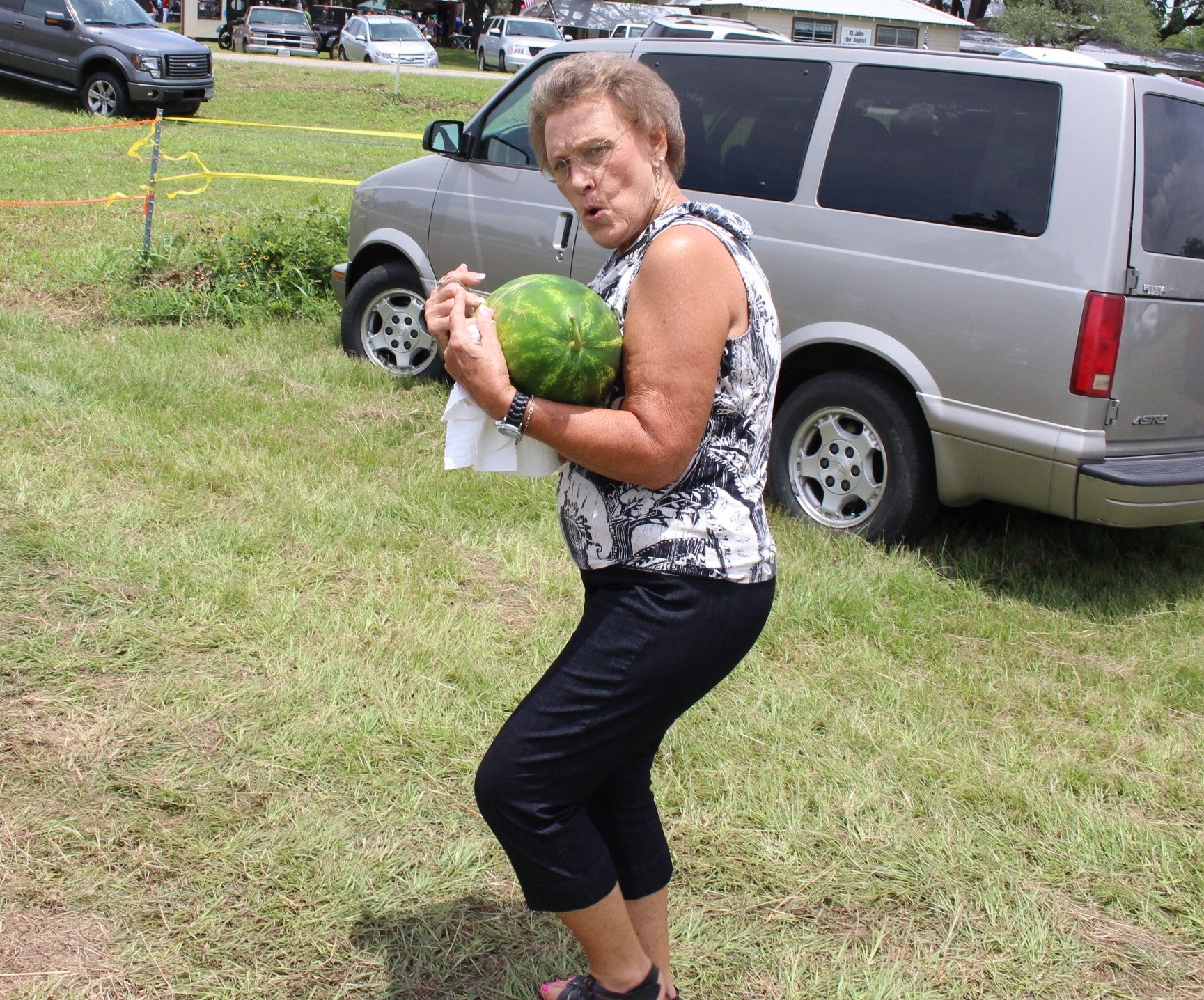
[540,971,680,1000]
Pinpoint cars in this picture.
[615,24,650,41]
[216,0,358,62]
[338,13,439,68]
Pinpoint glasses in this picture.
[540,119,641,184]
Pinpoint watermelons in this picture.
[471,273,622,405]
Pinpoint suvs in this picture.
[639,13,797,47]
[0,0,217,120]
[324,31,1204,548]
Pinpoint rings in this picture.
[439,276,445,287]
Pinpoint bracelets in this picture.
[515,395,535,445]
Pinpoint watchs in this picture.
[494,389,530,439]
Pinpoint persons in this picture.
[157,0,170,23]
[295,0,313,26]
[423,49,782,1000]
[366,9,473,49]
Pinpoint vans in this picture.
[477,13,575,77]
